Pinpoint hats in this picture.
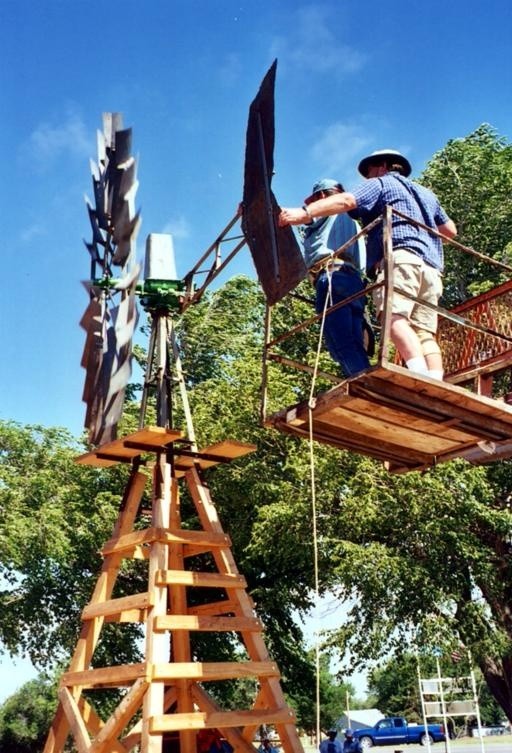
[358,150,411,176]
[305,179,343,205]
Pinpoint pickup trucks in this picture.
[353,716,445,749]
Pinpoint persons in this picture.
[319,727,343,752]
[298,178,371,378]
[277,147,459,382]
[343,729,363,752]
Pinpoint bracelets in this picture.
[302,205,311,218]
